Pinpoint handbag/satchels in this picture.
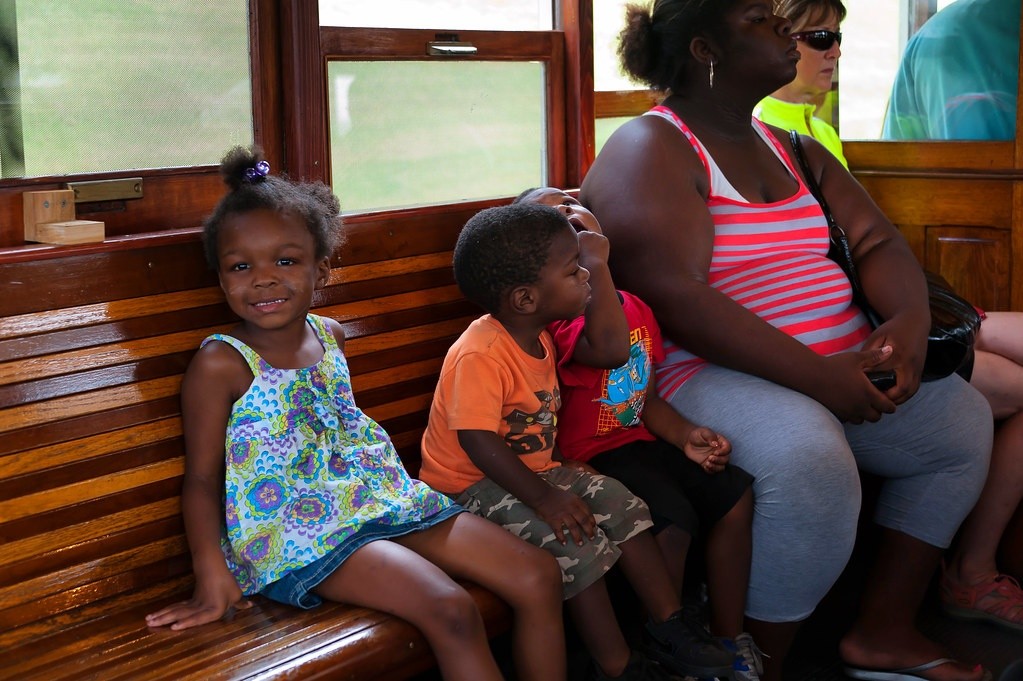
[789,130,981,382]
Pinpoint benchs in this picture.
[0,188,581,681]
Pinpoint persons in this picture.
[515,186,771,679]
[140,145,570,680]
[879,0,1019,141]
[752,0,1023,627]
[574,0,991,681]
[419,203,726,681]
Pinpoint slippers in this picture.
[847,656,993,681]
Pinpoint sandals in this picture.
[937,557,1023,630]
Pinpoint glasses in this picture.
[790,30,842,51]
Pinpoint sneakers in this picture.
[703,621,771,681]
[583,639,672,681]
[639,600,734,679]
